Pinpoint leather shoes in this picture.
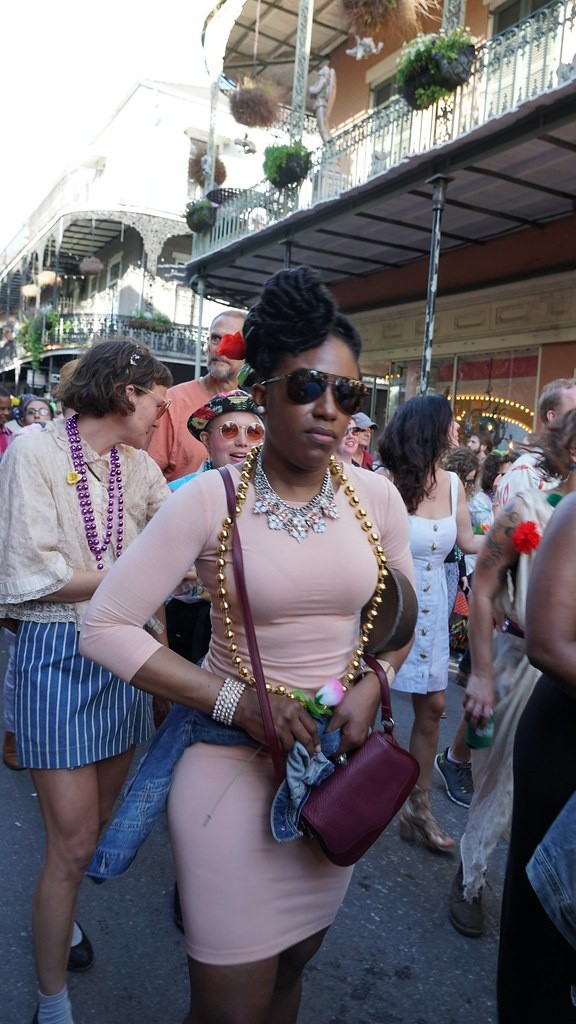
[68,920,94,972]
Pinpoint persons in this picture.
[345,35,383,61]
[0,338,199,1024]
[145,311,265,931]
[332,412,384,471]
[0,359,81,770]
[494,489,576,1024]
[309,66,335,143]
[374,393,489,853]
[440,377,576,934]
[80,264,416,1024]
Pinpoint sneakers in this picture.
[433,746,474,808]
[450,863,492,938]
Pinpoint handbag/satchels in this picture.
[300,730,420,867]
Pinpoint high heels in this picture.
[399,780,457,852]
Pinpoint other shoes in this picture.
[3,729,26,770]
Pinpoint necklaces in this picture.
[217,445,390,707]
[252,448,340,542]
[64,415,124,573]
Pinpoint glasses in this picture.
[205,421,263,442]
[130,384,171,420]
[343,426,362,436]
[260,368,369,415]
[25,408,51,416]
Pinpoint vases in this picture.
[186,207,216,231]
[402,44,476,111]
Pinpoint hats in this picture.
[187,390,264,443]
[352,412,380,430]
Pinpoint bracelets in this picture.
[212,678,246,725]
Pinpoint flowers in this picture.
[392,25,488,81]
[213,332,246,360]
[511,521,541,553]
[182,200,211,212]
[202,679,346,826]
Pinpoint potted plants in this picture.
[130,309,172,333]
[264,140,312,190]
[25,313,56,371]
